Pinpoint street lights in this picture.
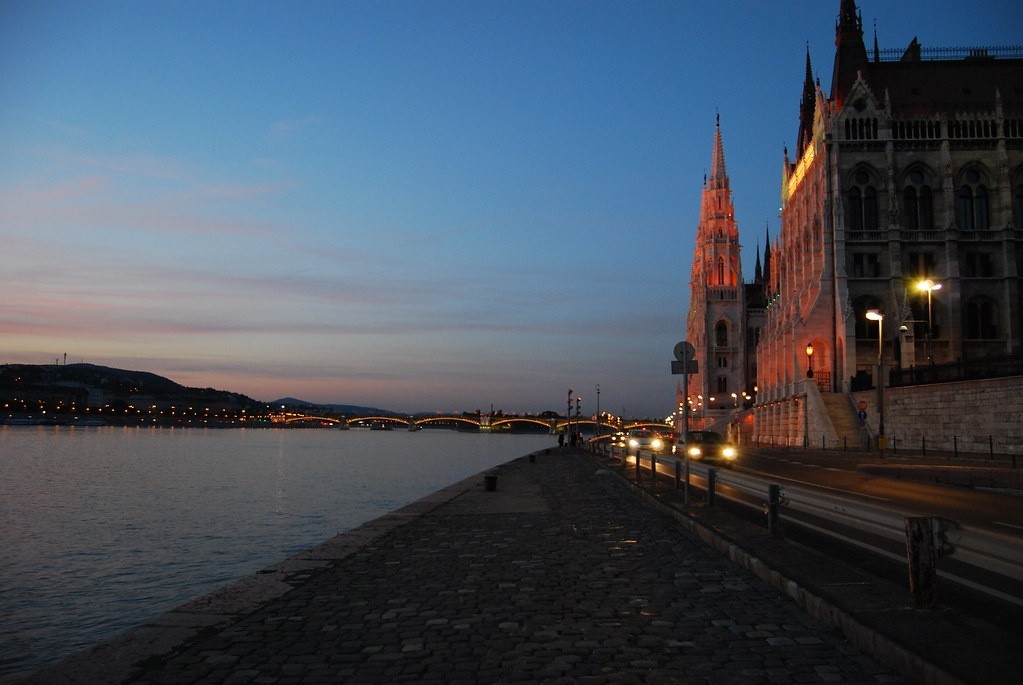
[805,342,814,378]
[567,389,573,439]
[916,278,941,385]
[665,386,758,428]
[596,383,603,439]
[866,309,893,459]
[575,397,582,440]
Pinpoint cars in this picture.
[612,429,627,443]
[659,431,674,441]
[624,428,663,455]
[671,430,735,471]
